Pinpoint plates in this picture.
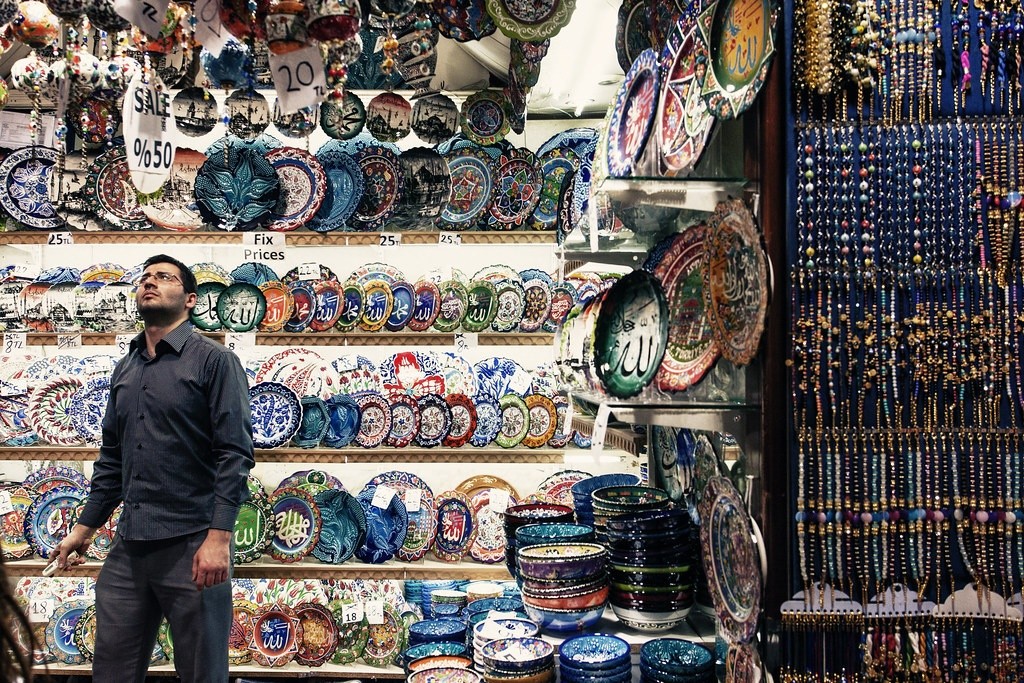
[0,348,591,448]
[7,600,420,668]
[0,262,621,334]
[0,127,598,231]
[487,0,577,42]
[606,0,781,178]
[459,91,511,145]
[0,467,592,564]
[551,195,772,393]
[698,476,763,645]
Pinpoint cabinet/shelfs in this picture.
[560,178,764,683]
[0,226,582,683]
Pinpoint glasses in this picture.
[132,273,186,287]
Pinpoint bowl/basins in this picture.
[403,618,481,683]
[501,472,699,632]
[467,596,539,680]
[406,580,458,620]
[459,580,521,602]
[429,590,469,620]
[557,633,714,683]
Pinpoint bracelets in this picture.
[780,0,1024,683]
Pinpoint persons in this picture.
[0,547,51,683]
[49,254,256,683]
